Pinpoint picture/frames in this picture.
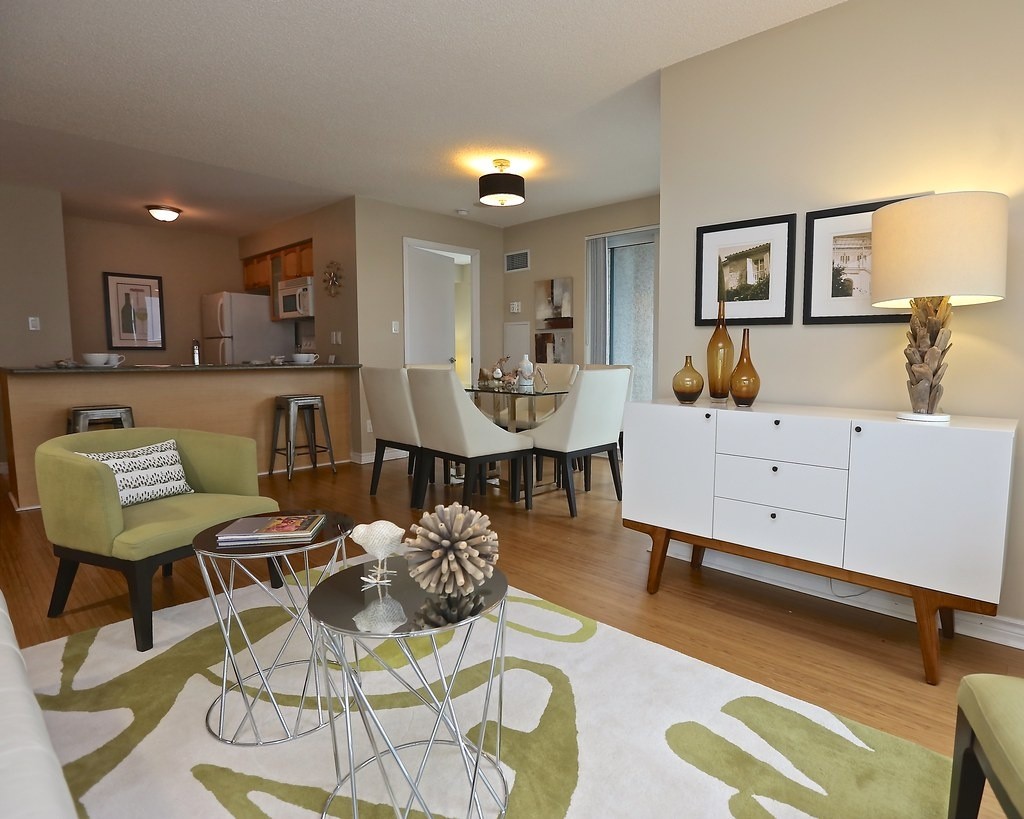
[102,272,166,350]
[803,195,925,325]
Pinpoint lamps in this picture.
[146,205,183,222]
[871,191,1009,422]
[478,159,526,206]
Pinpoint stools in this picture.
[66,405,135,434]
[269,394,337,482]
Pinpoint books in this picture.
[215,515,326,542]
[215,520,327,549]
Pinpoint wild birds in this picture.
[492,368,502,379]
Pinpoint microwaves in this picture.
[277,276,315,320]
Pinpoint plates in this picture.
[78,364,115,368]
[290,362,314,365]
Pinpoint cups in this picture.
[308,354,319,362]
[108,354,126,368]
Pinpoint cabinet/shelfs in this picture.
[622,396,1019,686]
[244,243,314,322]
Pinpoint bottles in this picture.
[518,386,533,394]
[519,354,533,385]
[707,300,734,403]
[672,356,704,404]
[728,328,761,407]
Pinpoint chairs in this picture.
[361,363,634,517]
[947,673,1024,819]
[35,427,284,652]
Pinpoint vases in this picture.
[695,213,797,326]
[673,300,760,408]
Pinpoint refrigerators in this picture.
[201,292,296,365]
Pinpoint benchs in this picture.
[0,589,78,818]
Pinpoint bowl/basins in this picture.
[271,356,285,365]
[81,353,108,365]
[292,354,308,362]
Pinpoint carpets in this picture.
[20,554,952,818]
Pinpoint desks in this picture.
[465,385,569,433]
[307,556,508,819]
[192,507,362,747]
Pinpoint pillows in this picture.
[74,439,194,509]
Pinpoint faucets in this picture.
[192,337,201,367]
